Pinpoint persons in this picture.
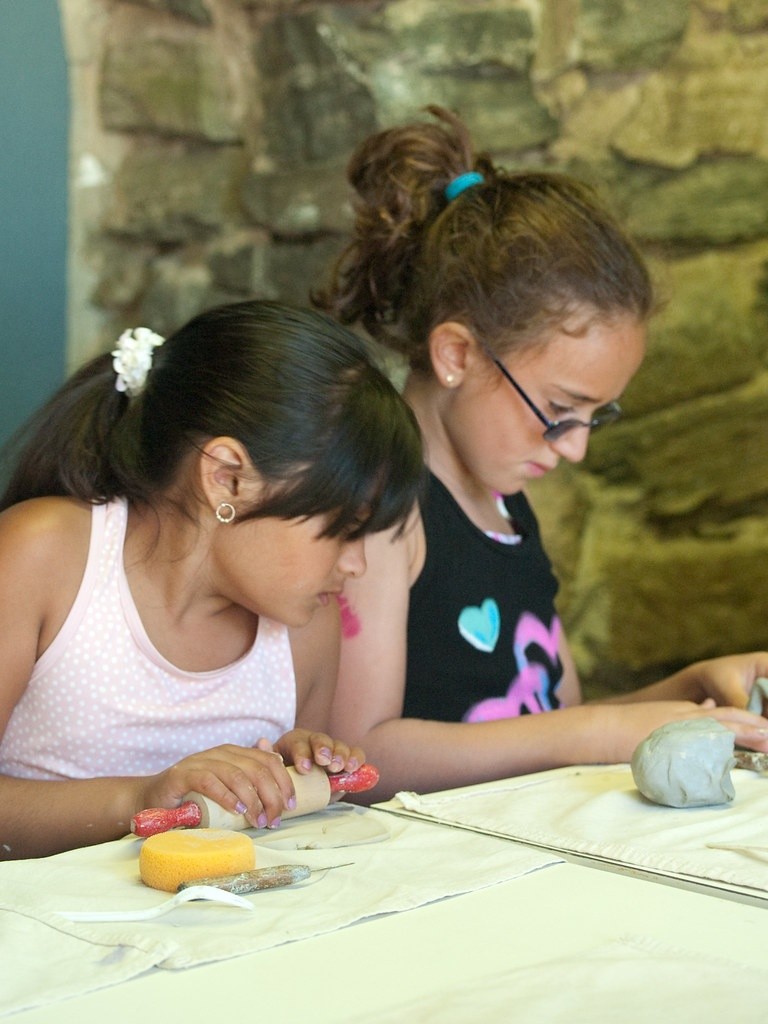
[0,301,424,860]
[303,104,768,802]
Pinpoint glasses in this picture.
[477,333,620,444]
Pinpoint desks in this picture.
[0,752,768,1024]
